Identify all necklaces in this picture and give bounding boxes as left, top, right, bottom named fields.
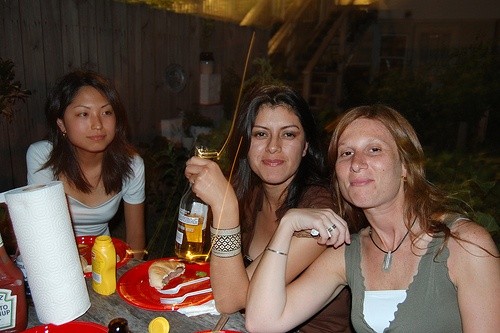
left=369, top=214, right=417, bottom=271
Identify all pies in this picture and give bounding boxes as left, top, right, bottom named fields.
left=148, top=261, right=185, bottom=289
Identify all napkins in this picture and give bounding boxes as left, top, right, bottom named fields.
left=177, top=299, right=221, bottom=317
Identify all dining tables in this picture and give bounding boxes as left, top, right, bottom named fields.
left=25, top=259, right=248, bottom=333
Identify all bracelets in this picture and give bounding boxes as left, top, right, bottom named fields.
left=210, top=225, right=242, bottom=258
left=266, top=247, right=288, bottom=256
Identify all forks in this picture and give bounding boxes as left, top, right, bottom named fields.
left=160, top=287, right=213, bottom=304
left=157, top=276, right=211, bottom=294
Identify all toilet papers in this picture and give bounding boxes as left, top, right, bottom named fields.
left=0, top=180, right=92, bottom=326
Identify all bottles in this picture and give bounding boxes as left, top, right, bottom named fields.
left=91, top=235, right=116, bottom=296
left=200, top=52, right=215, bottom=74
left=174, top=143, right=220, bottom=265
left=0, top=231, right=28, bottom=333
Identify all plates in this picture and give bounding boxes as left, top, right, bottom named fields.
left=21, top=321, right=109, bottom=333
left=116, top=257, right=213, bottom=311
left=75, top=235, right=132, bottom=278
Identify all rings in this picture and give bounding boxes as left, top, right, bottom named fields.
left=328, top=225, right=336, bottom=231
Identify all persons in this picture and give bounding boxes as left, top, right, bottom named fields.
left=246, top=102, right=500, bottom=333
left=184, top=84, right=337, bottom=313
left=26, top=70, right=147, bottom=258
